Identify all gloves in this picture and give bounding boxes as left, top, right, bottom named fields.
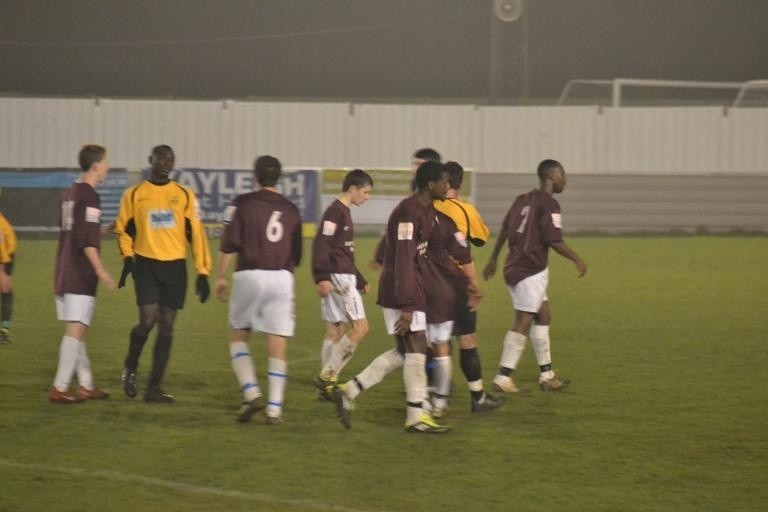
left=194, top=276, right=211, bottom=303
left=117, top=256, right=138, bottom=289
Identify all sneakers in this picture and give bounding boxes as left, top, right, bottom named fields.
left=471, top=392, right=507, bottom=416
left=491, top=375, right=530, bottom=395
left=48, top=385, right=86, bottom=406
left=333, top=383, right=359, bottom=430
left=429, top=391, right=449, bottom=418
left=121, top=366, right=139, bottom=398
left=404, top=414, right=453, bottom=434
left=312, top=373, right=340, bottom=401
left=76, top=385, right=109, bottom=401
left=2, top=336, right=15, bottom=344
left=143, top=388, right=175, bottom=404
left=539, top=376, right=571, bottom=392
left=263, top=411, right=287, bottom=427
left=234, top=393, right=266, bottom=425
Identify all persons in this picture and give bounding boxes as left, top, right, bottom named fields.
left=214, top=155, right=302, bottom=425
left=0, top=212, right=17, bottom=345
left=113, top=144, right=213, bottom=404
left=46, top=143, right=120, bottom=404
left=484, top=159, right=588, bottom=395
